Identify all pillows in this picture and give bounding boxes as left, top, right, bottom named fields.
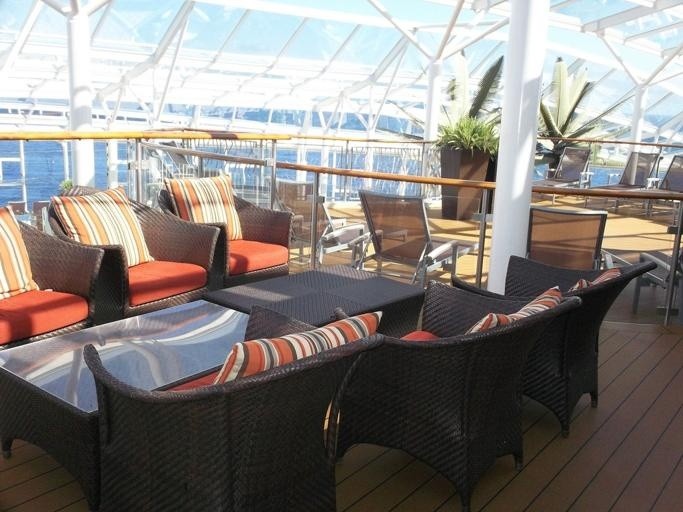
left=51, top=185, right=153, bottom=270
left=163, top=176, right=243, bottom=241
left=0, top=203, right=32, bottom=298
left=212, top=308, right=384, bottom=386
left=464, top=284, right=560, bottom=336
left=570, top=266, right=622, bottom=292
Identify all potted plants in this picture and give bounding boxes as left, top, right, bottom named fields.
left=434, top=111, right=500, bottom=220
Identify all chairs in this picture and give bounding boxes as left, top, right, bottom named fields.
left=532, top=146, right=594, bottom=202
left=0, top=221, right=105, bottom=350
left=644, top=155, right=683, bottom=220
left=584, top=152, right=662, bottom=213
left=48, top=191, right=222, bottom=323
left=273, top=184, right=363, bottom=268
left=359, top=188, right=478, bottom=284
left=453, top=256, right=658, bottom=424
left=159, top=183, right=293, bottom=287
left=340, top=278, right=579, bottom=512
left=631, top=200, right=683, bottom=324
left=85, top=301, right=381, bottom=512
left=528, top=207, right=613, bottom=273
